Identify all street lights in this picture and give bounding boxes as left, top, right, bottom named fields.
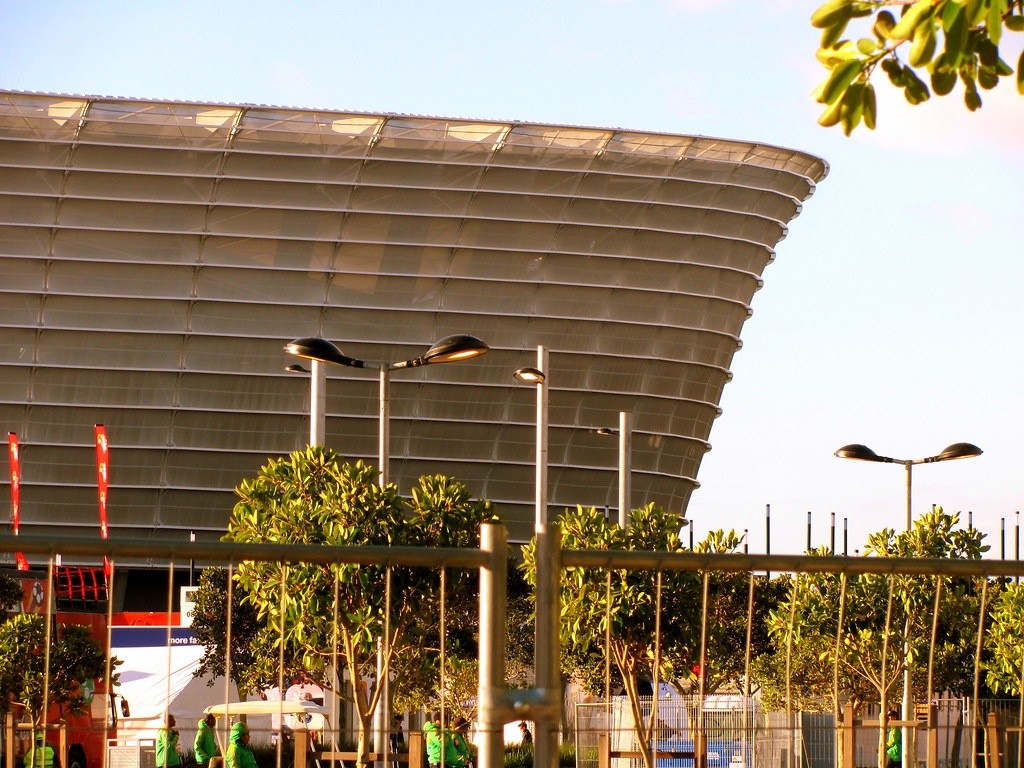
left=595, top=409, right=632, bottom=534
left=510, top=343, right=549, bottom=534
left=281, top=332, right=493, bottom=492
left=834, top=442, right=984, bottom=533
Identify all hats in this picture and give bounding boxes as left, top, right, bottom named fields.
left=887, top=711, right=897, bottom=716
left=36, top=734, right=43, bottom=740
left=451, top=717, right=467, bottom=731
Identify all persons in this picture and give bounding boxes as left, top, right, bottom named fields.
left=886, top=711, right=902, bottom=768
left=156, top=714, right=182, bottom=768
left=423, top=712, right=475, bottom=768
left=226, top=722, right=257, bottom=768
left=390, top=715, right=407, bottom=753
left=517, top=722, right=532, bottom=746
left=24, top=734, right=54, bottom=768
left=194, top=714, right=216, bottom=768
